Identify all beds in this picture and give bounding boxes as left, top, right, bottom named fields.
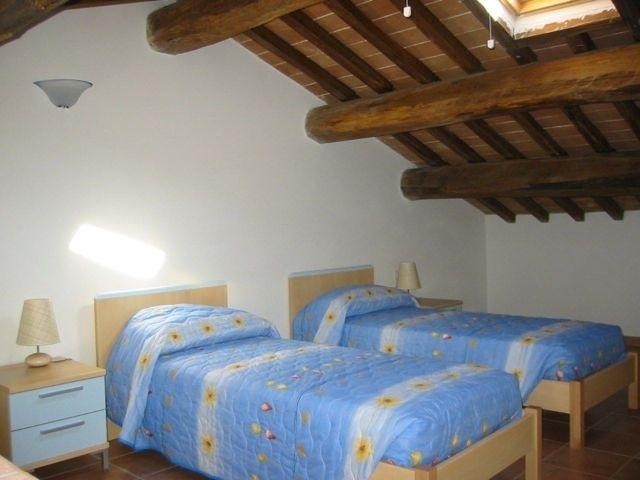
left=94, top=280, right=543, bottom=480
left=289, top=265, right=639, bottom=450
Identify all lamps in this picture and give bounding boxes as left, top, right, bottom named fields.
left=16, top=298, right=60, bottom=367
left=33, top=79, right=93, bottom=109
left=396, top=262, right=421, bottom=293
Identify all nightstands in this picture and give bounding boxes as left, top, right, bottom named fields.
left=416, top=298, right=463, bottom=311
left=0, top=357, right=110, bottom=472
